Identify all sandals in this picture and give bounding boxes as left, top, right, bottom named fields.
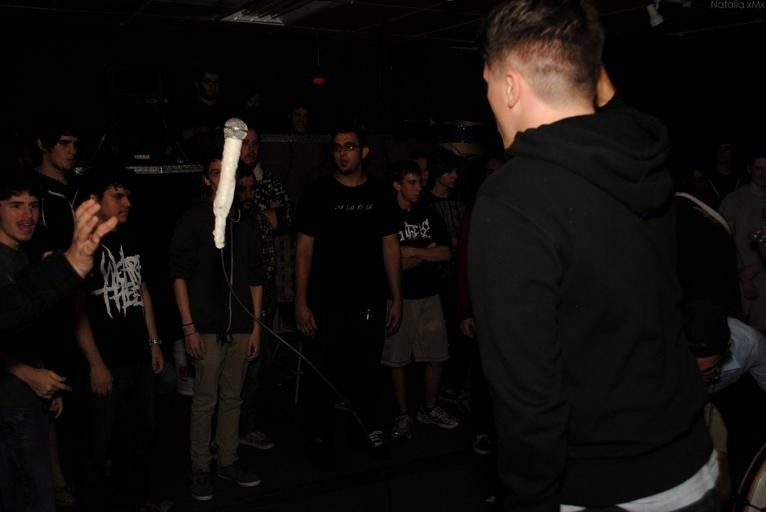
left=51, top=482, right=75, bottom=507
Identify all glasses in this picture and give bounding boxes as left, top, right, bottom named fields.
left=331, top=141, right=363, bottom=153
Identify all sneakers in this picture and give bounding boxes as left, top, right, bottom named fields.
left=439, top=388, right=459, bottom=404
left=209, top=441, right=239, bottom=464
left=190, top=468, right=214, bottom=501
left=416, top=405, right=460, bottom=429
left=239, top=432, right=274, bottom=450
left=390, top=413, right=413, bottom=440
left=215, top=460, right=262, bottom=487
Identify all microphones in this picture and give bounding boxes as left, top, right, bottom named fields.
left=213, top=118, right=248, bottom=250
